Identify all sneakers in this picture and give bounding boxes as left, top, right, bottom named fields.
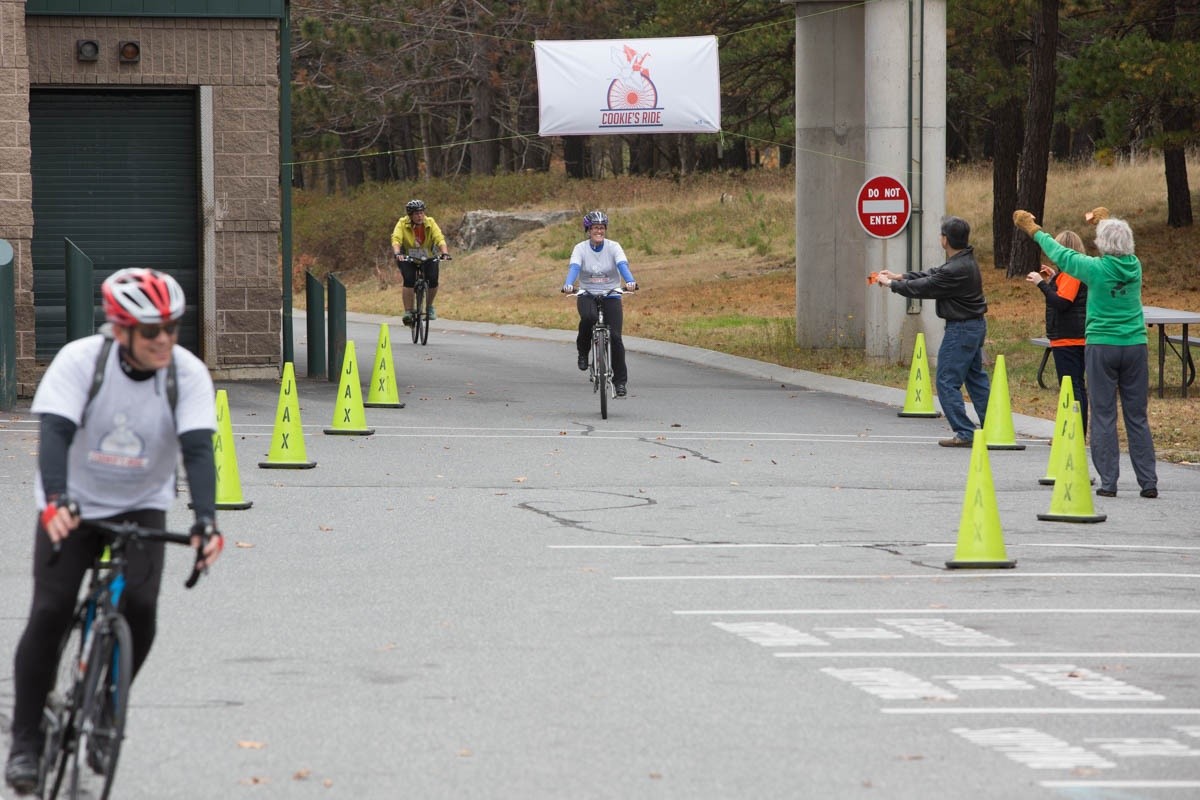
left=86, top=726, right=117, bottom=776
left=6, top=735, right=40, bottom=786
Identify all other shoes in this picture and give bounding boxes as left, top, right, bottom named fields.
left=616, top=383, right=627, bottom=399
left=426, top=306, right=435, bottom=319
left=578, top=351, right=588, bottom=371
left=1095, top=489, right=1115, bottom=497
left=401, top=313, right=413, bottom=326
left=939, top=437, right=973, bottom=448
left=1141, top=487, right=1158, bottom=498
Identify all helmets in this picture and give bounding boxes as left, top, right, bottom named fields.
left=583, top=211, right=608, bottom=226
left=101, top=267, right=186, bottom=325
left=405, top=200, right=426, bottom=211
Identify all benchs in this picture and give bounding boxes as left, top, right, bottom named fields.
left=1165, top=335, right=1200, bottom=387
left=1031, top=338, right=1051, bottom=389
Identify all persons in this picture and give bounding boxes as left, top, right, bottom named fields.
left=1025, top=230, right=1088, bottom=446
left=876, top=216, right=990, bottom=447
left=4, top=266, right=225, bottom=792
left=390, top=200, right=450, bottom=326
left=1012, top=207, right=1159, bottom=498
left=562, top=211, right=636, bottom=396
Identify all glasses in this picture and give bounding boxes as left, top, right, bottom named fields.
left=116, top=323, right=182, bottom=339
left=589, top=226, right=606, bottom=232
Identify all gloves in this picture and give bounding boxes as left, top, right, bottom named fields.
left=1014, top=210, right=1041, bottom=237
left=1089, top=207, right=1111, bottom=224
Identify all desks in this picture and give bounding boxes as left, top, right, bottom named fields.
left=1141, top=305, right=1200, bottom=399
